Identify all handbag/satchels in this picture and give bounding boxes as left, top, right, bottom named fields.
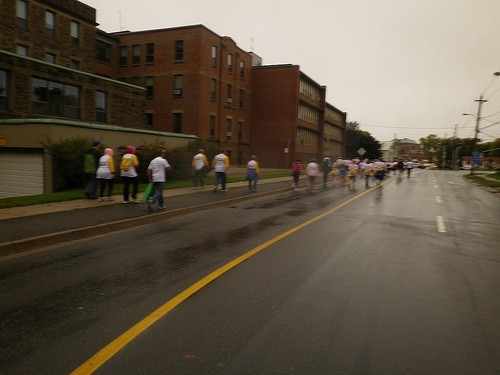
left=293, top=171, right=299, bottom=178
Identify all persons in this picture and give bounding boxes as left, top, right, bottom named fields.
left=147, top=150, right=171, bottom=210
left=246, top=155, right=259, bottom=192
left=83, top=139, right=103, bottom=200
left=212, top=148, right=230, bottom=192
left=192, top=148, right=209, bottom=190
left=322, top=156, right=413, bottom=191
left=119, top=146, right=140, bottom=204
left=291, top=159, right=302, bottom=187
left=96, top=147, right=116, bottom=202
left=305, top=159, right=319, bottom=190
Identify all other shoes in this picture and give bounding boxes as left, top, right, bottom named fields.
left=98, top=199, right=103, bottom=202
left=221, top=189, right=229, bottom=192
left=249, top=190, right=251, bottom=192
left=85, top=192, right=91, bottom=199
left=108, top=197, right=113, bottom=200
left=131, top=197, right=136, bottom=202
left=123, top=201, right=129, bottom=203
left=157, top=206, right=166, bottom=209
left=148, top=200, right=154, bottom=210
left=252, top=189, right=256, bottom=191
left=92, top=196, right=98, bottom=199
left=201, top=186, right=206, bottom=188
left=193, top=186, right=199, bottom=190
left=213, top=186, right=218, bottom=192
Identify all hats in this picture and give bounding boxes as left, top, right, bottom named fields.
left=93, top=140, right=100, bottom=146
left=127, top=146, right=137, bottom=154
left=104, top=148, right=112, bottom=155
